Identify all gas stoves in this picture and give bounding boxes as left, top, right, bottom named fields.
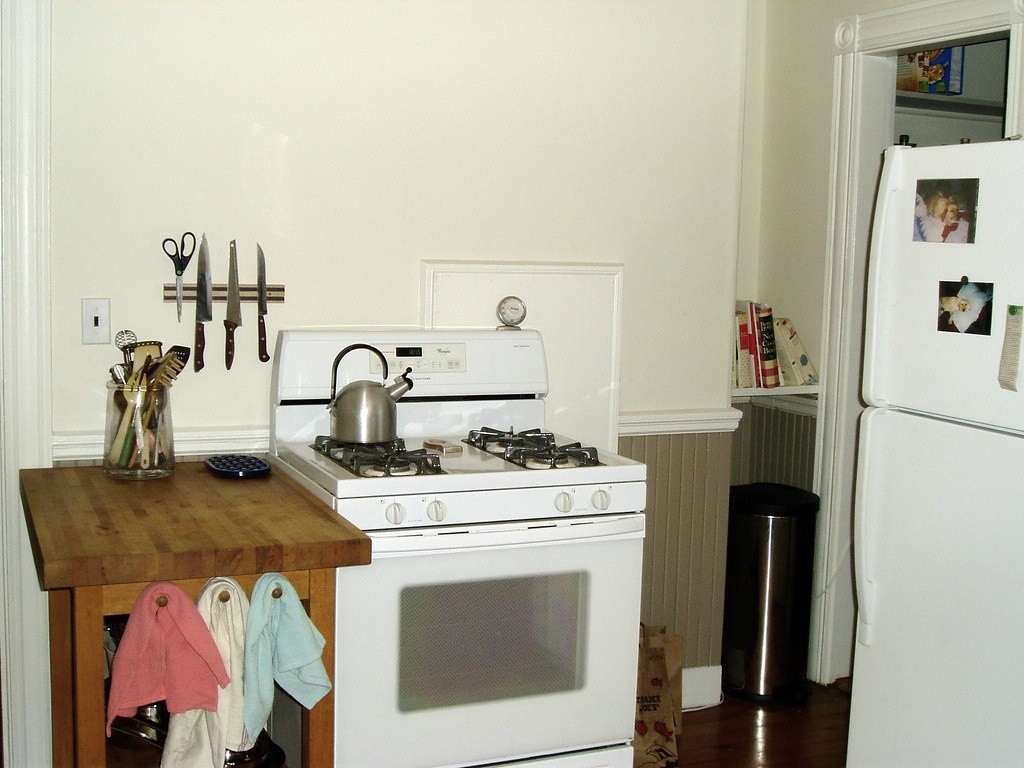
left=269, top=329, right=647, bottom=530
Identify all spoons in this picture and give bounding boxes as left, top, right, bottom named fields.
left=153, top=389, right=167, bottom=467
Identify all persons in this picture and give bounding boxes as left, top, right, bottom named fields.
left=915, top=190, right=968, bottom=243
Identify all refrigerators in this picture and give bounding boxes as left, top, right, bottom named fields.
left=846, top=139, right=1024, bottom=768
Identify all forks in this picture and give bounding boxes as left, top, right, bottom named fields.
left=108, top=371, right=147, bottom=465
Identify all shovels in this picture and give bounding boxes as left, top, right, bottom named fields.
left=117, top=340, right=190, bottom=466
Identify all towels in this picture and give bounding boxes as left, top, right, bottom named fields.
left=106, top=573, right=331, bottom=768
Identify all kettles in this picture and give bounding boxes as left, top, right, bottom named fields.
left=325, top=344, right=413, bottom=445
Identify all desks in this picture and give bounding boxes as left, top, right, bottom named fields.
left=19, top=461, right=372, bottom=768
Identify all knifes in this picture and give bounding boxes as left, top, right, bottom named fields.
left=257, top=243, right=270, bottom=363
left=194, top=232, right=213, bottom=373
left=223, top=239, right=242, bottom=370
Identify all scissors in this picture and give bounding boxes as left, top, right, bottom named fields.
left=162, top=232, right=197, bottom=324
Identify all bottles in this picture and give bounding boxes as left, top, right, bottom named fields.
left=102, top=380, right=175, bottom=483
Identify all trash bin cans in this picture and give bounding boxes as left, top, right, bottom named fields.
left=724, top=482, right=820, bottom=705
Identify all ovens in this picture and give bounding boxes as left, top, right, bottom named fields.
left=336, top=513, right=645, bottom=768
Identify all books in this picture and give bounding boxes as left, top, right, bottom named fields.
left=896, top=46, right=965, bottom=95
left=732, top=302, right=818, bottom=388
